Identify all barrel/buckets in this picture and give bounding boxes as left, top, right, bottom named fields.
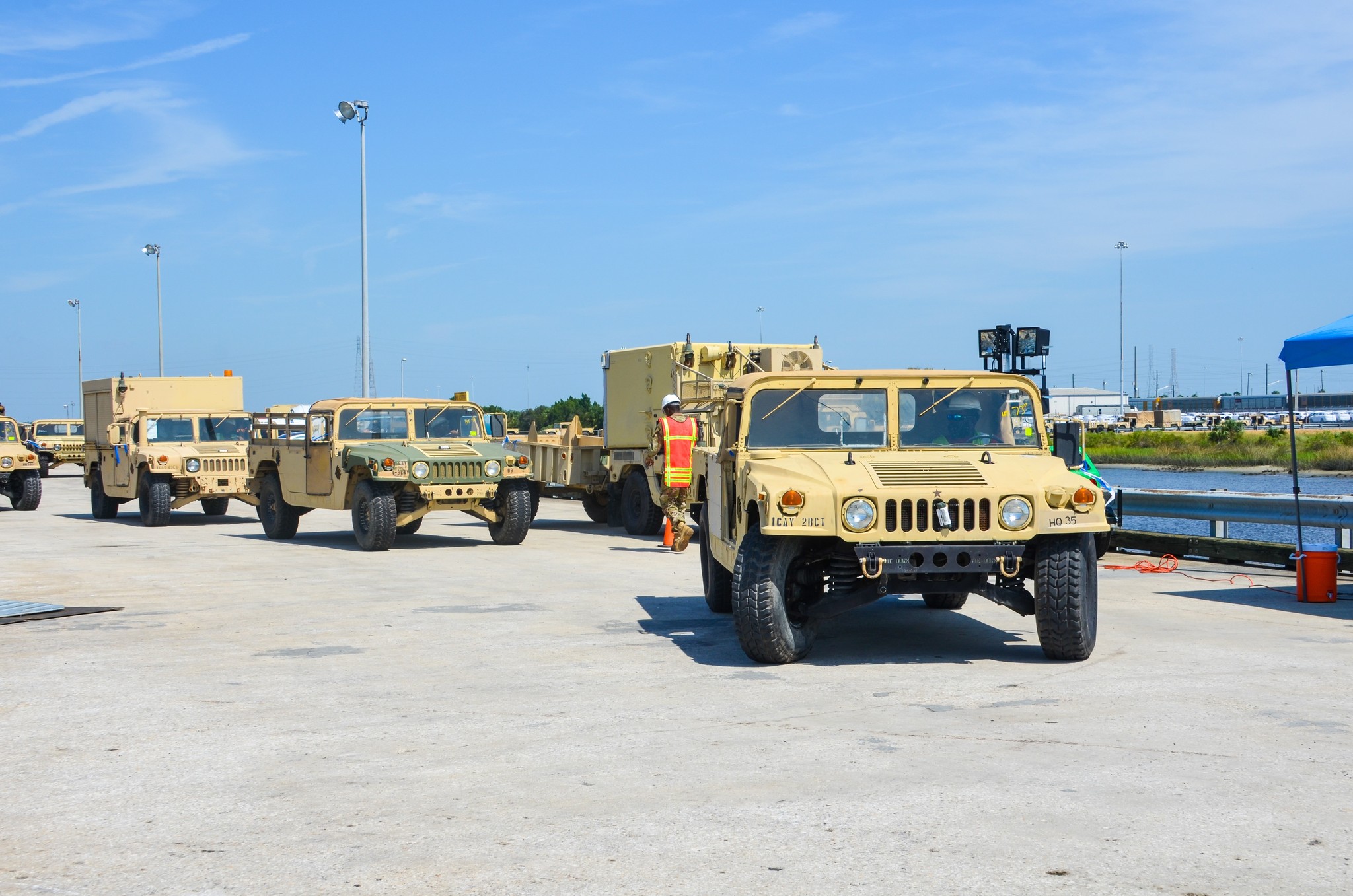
left=1289, top=543, right=1341, bottom=602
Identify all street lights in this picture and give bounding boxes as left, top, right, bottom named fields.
left=334, top=99, right=370, bottom=403
left=755, top=307, right=764, bottom=344
left=425, top=389, right=429, bottom=398
left=1053, top=385, right=1058, bottom=389
left=63, top=405, right=69, bottom=419
left=1247, top=371, right=1254, bottom=395
left=1104, top=379, right=1107, bottom=391
left=1114, top=240, right=1129, bottom=413
left=1320, top=369, right=1326, bottom=391
left=67, top=299, right=82, bottom=415
left=399, top=358, right=407, bottom=399
left=436, top=386, right=440, bottom=399
left=470, top=377, right=475, bottom=401
left=1237, top=336, right=1245, bottom=395
left=141, top=243, right=165, bottom=378
left=1204, top=366, right=1209, bottom=400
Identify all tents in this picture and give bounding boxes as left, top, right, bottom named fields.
left=1277, top=315, right=1353, bottom=602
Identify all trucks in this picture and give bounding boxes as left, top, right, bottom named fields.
left=0, top=402, right=42, bottom=512
left=25, top=418, right=85, bottom=478
left=689, top=366, right=1112, bottom=665
left=491, top=333, right=824, bottom=538
left=80, top=370, right=272, bottom=529
left=242, top=398, right=538, bottom=553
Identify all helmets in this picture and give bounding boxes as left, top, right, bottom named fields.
left=224, top=418, right=236, bottom=426
left=944, top=392, right=982, bottom=411
left=431, top=416, right=449, bottom=429
left=661, top=394, right=681, bottom=412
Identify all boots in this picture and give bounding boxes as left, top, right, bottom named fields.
left=670, top=522, right=694, bottom=552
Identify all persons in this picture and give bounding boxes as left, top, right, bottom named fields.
left=430, top=415, right=460, bottom=438
left=644, top=394, right=698, bottom=552
left=363, top=421, right=390, bottom=433
left=212, top=417, right=244, bottom=440
left=930, top=392, right=1002, bottom=446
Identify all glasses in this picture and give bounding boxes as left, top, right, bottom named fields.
left=662, top=411, right=666, bottom=415
left=947, top=414, right=976, bottom=421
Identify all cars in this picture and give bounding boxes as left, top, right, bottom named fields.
left=1012, top=410, right=1353, bottom=429
